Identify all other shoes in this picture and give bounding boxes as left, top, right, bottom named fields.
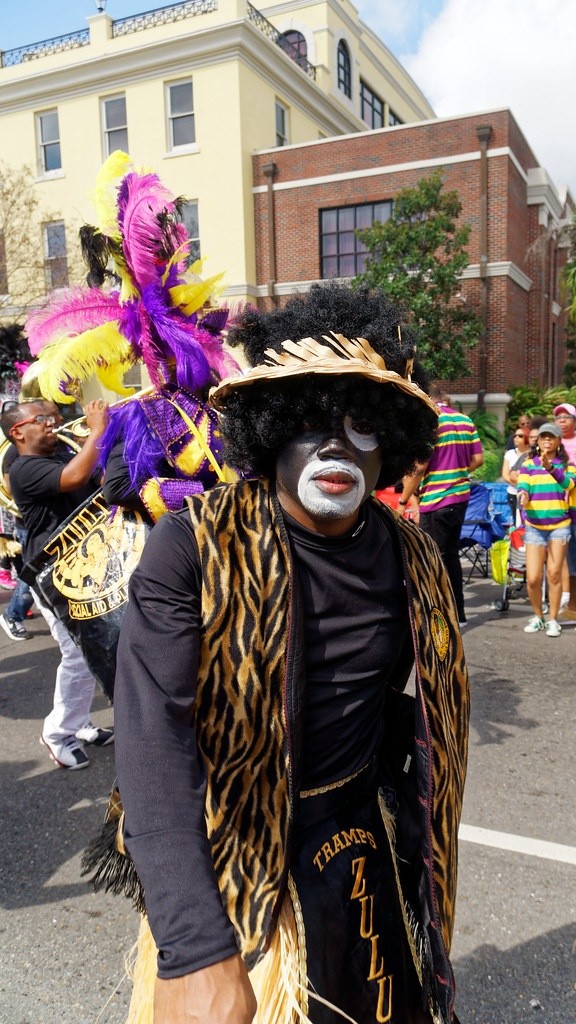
left=557, top=606, right=576, bottom=624
left=459, top=616, right=469, bottom=628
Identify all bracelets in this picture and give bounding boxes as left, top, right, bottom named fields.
left=398, top=497, right=408, bottom=505
left=546, top=464, right=554, bottom=472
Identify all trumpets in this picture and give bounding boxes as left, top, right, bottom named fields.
left=49, top=368, right=167, bottom=439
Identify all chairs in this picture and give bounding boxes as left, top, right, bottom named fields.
left=459, top=482, right=490, bottom=584
left=487, top=483, right=515, bottom=529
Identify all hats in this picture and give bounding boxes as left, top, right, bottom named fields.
left=206, top=292, right=440, bottom=489
left=537, top=423, right=562, bottom=442
left=552, top=402, right=576, bottom=419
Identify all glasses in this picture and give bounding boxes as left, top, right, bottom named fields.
left=552, top=415, right=575, bottom=420
left=514, top=433, right=525, bottom=438
left=7, top=414, right=54, bottom=435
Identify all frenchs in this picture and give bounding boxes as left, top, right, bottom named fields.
left=0, top=332, right=82, bottom=519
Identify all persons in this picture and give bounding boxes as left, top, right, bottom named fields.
left=502, top=403, right=576, bottom=636
left=398, top=383, right=483, bottom=626
left=0, top=398, right=115, bottom=770
left=81, top=284, right=471, bottom=1024
left=0, top=400, right=34, bottom=641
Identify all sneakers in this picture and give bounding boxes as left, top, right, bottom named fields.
left=38, top=732, right=89, bottom=770
left=524, top=617, right=543, bottom=633
left=75, top=719, right=115, bottom=745
left=0, top=611, right=30, bottom=640
left=545, top=619, right=560, bottom=637
left=0, top=569, right=17, bottom=590
